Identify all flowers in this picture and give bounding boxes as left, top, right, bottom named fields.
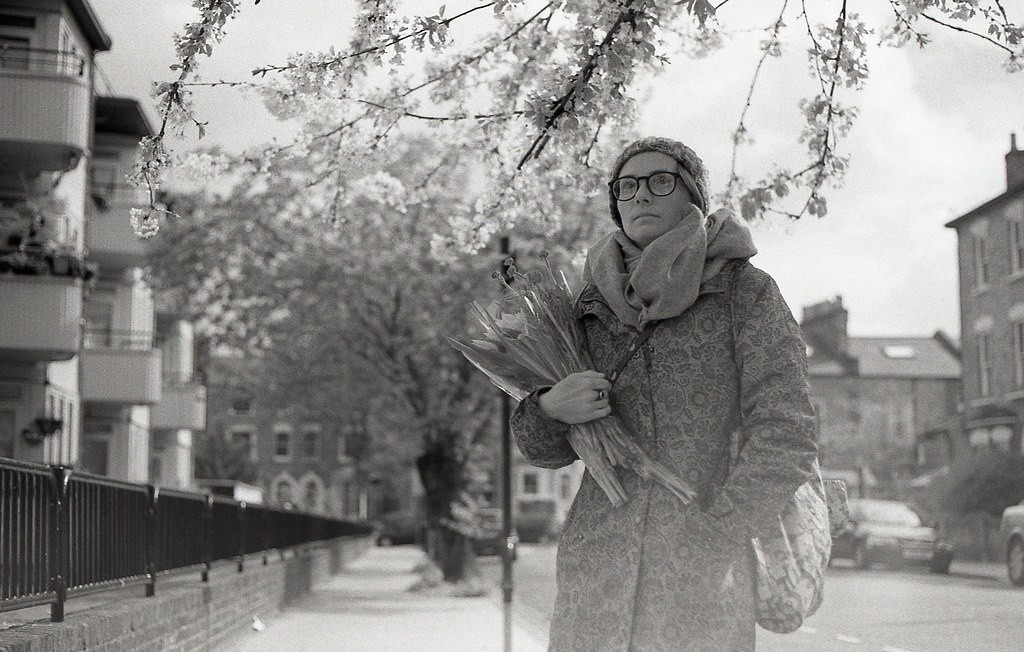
left=443, top=250, right=698, bottom=505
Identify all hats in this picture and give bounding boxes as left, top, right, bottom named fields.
left=608, top=136, right=710, bottom=229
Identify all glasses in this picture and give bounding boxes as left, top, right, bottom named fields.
left=612, top=171, right=680, bottom=201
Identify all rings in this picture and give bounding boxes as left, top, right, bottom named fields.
left=595, top=389, right=605, bottom=401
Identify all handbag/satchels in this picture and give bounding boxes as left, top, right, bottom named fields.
left=730, top=424, right=832, bottom=635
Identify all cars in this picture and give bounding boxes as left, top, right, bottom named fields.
left=377, top=513, right=430, bottom=548
left=999, top=500, right=1024, bottom=586
left=473, top=509, right=517, bottom=562
left=829, top=499, right=953, bottom=573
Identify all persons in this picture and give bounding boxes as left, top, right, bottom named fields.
left=510, top=136, right=818, bottom=650
